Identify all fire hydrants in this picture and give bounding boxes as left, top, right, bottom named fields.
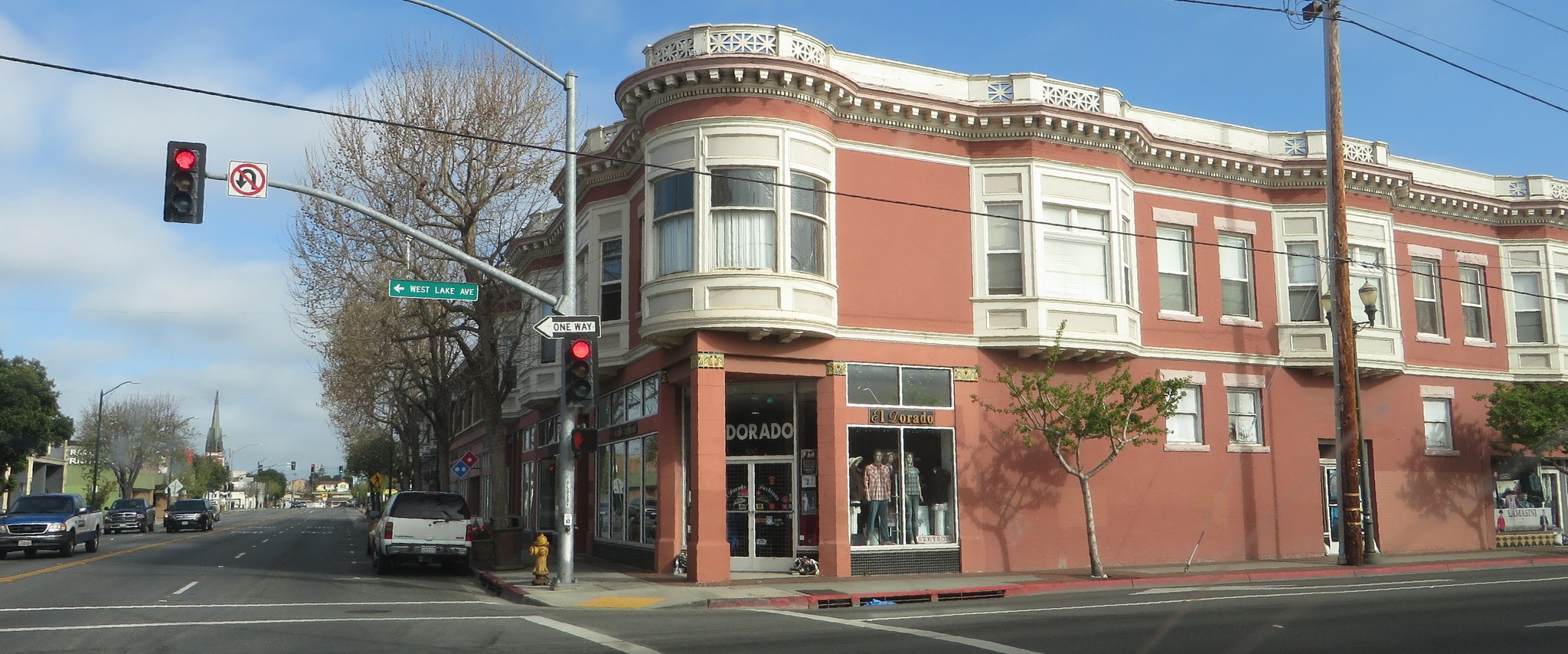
left=528, top=532, right=550, bottom=586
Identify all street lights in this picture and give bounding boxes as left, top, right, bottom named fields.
left=207, top=435, right=230, bottom=501
left=90, top=380, right=142, bottom=512
left=1317, top=277, right=1382, bottom=566
left=166, top=416, right=202, bottom=508
left=228, top=443, right=263, bottom=511
left=255, top=457, right=270, bottom=510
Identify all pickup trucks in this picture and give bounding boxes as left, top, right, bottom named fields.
left=0, top=492, right=105, bottom=562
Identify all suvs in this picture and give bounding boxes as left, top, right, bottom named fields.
left=103, top=496, right=156, bottom=535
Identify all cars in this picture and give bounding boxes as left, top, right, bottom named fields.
left=366, top=489, right=473, bottom=576
left=289, top=500, right=307, bottom=509
left=163, top=497, right=221, bottom=533
left=344, top=500, right=358, bottom=507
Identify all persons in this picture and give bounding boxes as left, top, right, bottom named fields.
left=1497, top=509, right=1505, bottom=532
left=1540, top=509, right=1549, bottom=529
left=1518, top=493, right=1533, bottom=507
left=849, top=448, right=923, bottom=545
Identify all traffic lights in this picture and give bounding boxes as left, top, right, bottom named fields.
left=571, top=428, right=598, bottom=452
left=311, top=464, right=315, bottom=472
left=570, top=339, right=594, bottom=400
left=163, top=141, right=207, bottom=225
left=339, top=465, right=343, bottom=473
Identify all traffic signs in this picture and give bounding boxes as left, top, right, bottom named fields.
left=532, top=314, right=602, bottom=340
